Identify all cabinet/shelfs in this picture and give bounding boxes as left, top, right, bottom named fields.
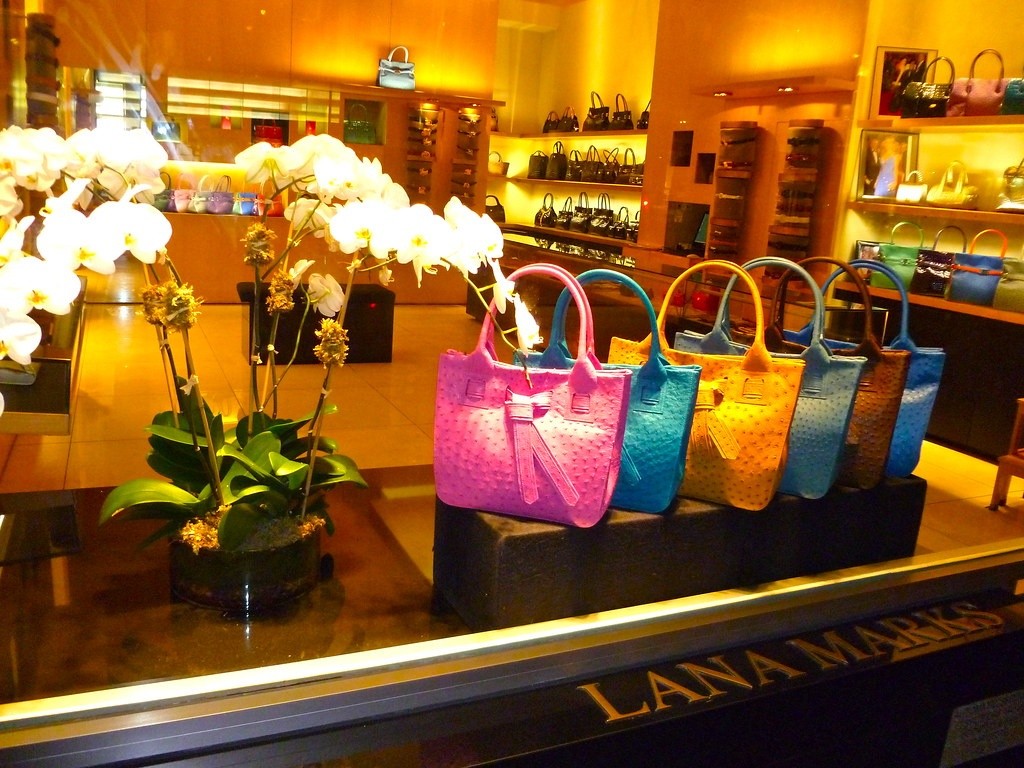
left=832, top=114, right=1024, bottom=465
left=497, top=129, right=649, bottom=250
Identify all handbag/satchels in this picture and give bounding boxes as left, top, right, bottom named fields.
left=155, top=171, right=285, bottom=217
left=997, top=158, right=1024, bottom=210
left=528, top=91, right=651, bottom=243
left=925, top=160, right=979, bottom=210
left=253, top=119, right=283, bottom=148
left=764, top=256, right=910, bottom=489
left=675, top=257, right=867, bottom=499
left=380, top=45, right=416, bottom=91
left=900, top=56, right=956, bottom=117
left=784, top=259, right=945, bottom=480
left=485, top=195, right=505, bottom=222
left=517, top=269, right=702, bottom=513
left=947, top=49, right=1012, bottom=116
left=434, top=263, right=633, bottom=527
left=344, top=103, right=375, bottom=144
left=608, top=260, right=806, bottom=511
left=895, top=171, right=928, bottom=205
left=1001, top=66, right=1024, bottom=114
left=871, top=220, right=1023, bottom=307
left=488, top=151, right=509, bottom=175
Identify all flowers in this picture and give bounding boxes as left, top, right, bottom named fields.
left=0, top=117, right=544, bottom=565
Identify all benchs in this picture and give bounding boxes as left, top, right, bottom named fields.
left=236, top=282, right=395, bottom=367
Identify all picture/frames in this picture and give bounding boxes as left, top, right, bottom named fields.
left=855, top=129, right=920, bottom=204
left=867, top=46, right=938, bottom=119
left=855, top=239, right=895, bottom=284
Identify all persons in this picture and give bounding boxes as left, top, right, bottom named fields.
left=863, top=134, right=907, bottom=197
left=882, top=54, right=926, bottom=112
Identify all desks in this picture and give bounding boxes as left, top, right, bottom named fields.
left=430, top=473, right=927, bottom=629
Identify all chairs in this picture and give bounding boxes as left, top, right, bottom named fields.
left=988, top=397, right=1024, bottom=511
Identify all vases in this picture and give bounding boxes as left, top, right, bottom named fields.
left=167, top=509, right=324, bottom=613
